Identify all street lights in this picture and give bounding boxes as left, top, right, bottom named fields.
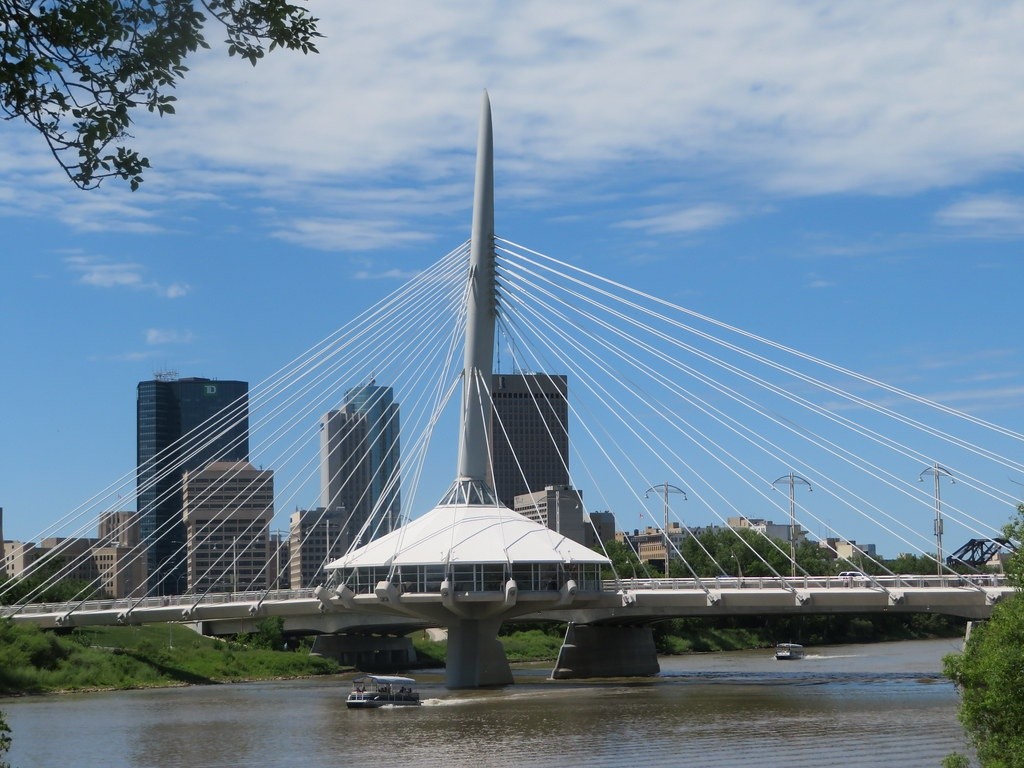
left=644, top=482, right=687, bottom=579
left=918, top=463, right=956, bottom=575
left=771, top=472, right=813, bottom=581
left=212, top=535, right=255, bottom=602
left=536, top=490, right=580, bottom=590
left=255, top=528, right=301, bottom=590
left=305, top=518, right=351, bottom=583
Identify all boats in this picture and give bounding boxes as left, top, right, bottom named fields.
left=774, top=643, right=804, bottom=659
left=344, top=675, right=422, bottom=708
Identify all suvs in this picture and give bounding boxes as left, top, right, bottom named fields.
left=838, top=572, right=873, bottom=587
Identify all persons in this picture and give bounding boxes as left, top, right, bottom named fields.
left=355, top=687, right=362, bottom=692
left=361, top=686, right=367, bottom=693
left=386, top=684, right=412, bottom=694
